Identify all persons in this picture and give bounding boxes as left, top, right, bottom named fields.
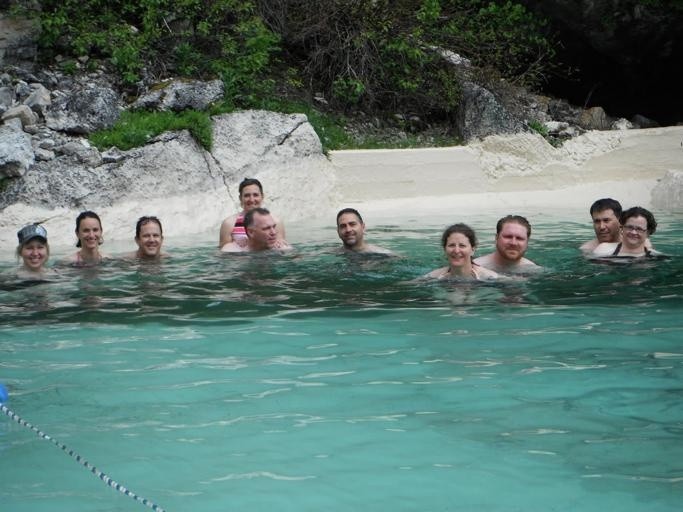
left=3, top=223, right=58, bottom=277
left=220, top=177, right=285, bottom=243
left=126, top=214, right=170, bottom=261
left=318, top=209, right=391, bottom=251
left=579, top=197, right=623, bottom=251
left=473, top=214, right=536, bottom=270
left=222, top=207, right=290, bottom=251
left=597, top=206, right=662, bottom=256
left=413, top=224, right=500, bottom=280
left=56, top=210, right=112, bottom=265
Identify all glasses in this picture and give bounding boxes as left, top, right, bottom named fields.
left=139, top=216, right=159, bottom=225
left=625, top=224, right=648, bottom=233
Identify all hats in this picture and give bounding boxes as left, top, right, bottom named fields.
left=17, top=225, right=48, bottom=244
